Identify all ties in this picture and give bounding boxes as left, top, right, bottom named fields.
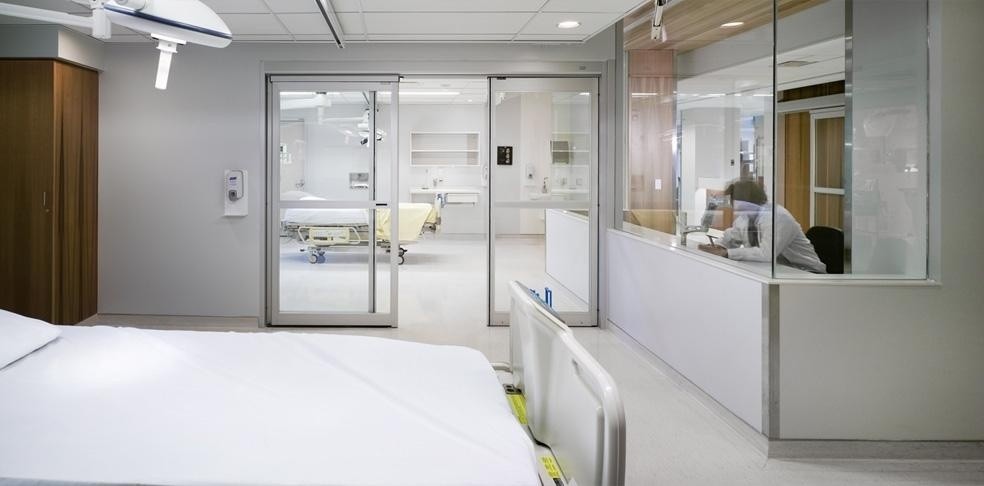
left=748, top=219, right=760, bottom=248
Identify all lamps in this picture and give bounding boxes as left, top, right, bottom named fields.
left=77, top=0, right=237, bottom=92
left=353, top=119, right=383, bottom=149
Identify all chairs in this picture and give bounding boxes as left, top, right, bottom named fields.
left=784, top=223, right=845, bottom=275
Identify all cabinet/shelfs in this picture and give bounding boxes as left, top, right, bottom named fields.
left=0, top=58, right=100, bottom=325
left=406, top=129, right=484, bottom=172
left=550, top=130, right=590, bottom=167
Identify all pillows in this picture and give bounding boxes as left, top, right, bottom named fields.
left=0, top=308, right=63, bottom=372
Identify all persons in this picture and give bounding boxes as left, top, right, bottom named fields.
left=698, top=182, right=828, bottom=274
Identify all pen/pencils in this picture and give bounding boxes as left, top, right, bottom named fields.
left=709, top=235, right=715, bottom=246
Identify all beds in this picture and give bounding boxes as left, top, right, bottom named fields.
left=1, top=278, right=631, bottom=486
left=278, top=187, right=439, bottom=264
